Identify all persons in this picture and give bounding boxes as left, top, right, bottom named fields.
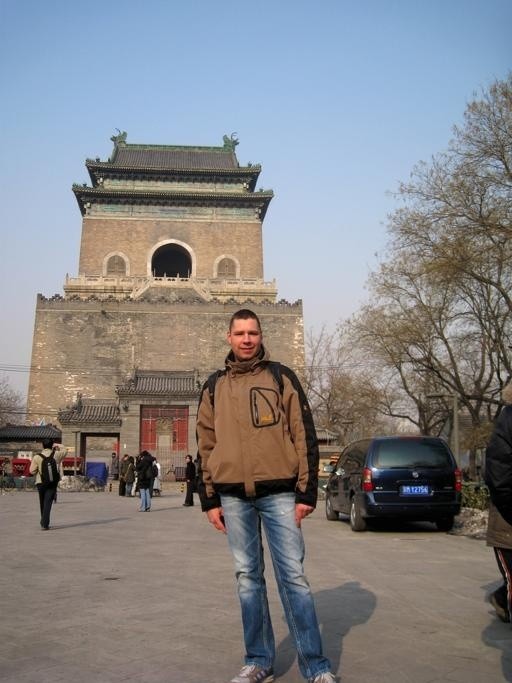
left=29, top=437, right=67, bottom=530
left=111, top=452, right=120, bottom=479
left=197, top=309, right=339, bottom=683
left=183, top=454, right=195, bottom=506
left=486, top=378, right=512, bottom=622
left=118, top=449, right=162, bottom=512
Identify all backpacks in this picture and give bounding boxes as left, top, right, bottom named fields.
left=36, top=448, right=59, bottom=487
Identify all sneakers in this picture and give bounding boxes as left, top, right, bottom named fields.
left=39, top=524, right=50, bottom=530
left=488, top=590, right=510, bottom=622
left=308, top=672, right=337, bottom=683
left=118, top=493, right=195, bottom=513
left=230, top=663, right=274, bottom=683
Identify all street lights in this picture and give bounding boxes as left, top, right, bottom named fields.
left=427, top=393, right=460, bottom=468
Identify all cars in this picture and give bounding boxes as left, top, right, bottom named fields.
left=326, top=435, right=463, bottom=532
